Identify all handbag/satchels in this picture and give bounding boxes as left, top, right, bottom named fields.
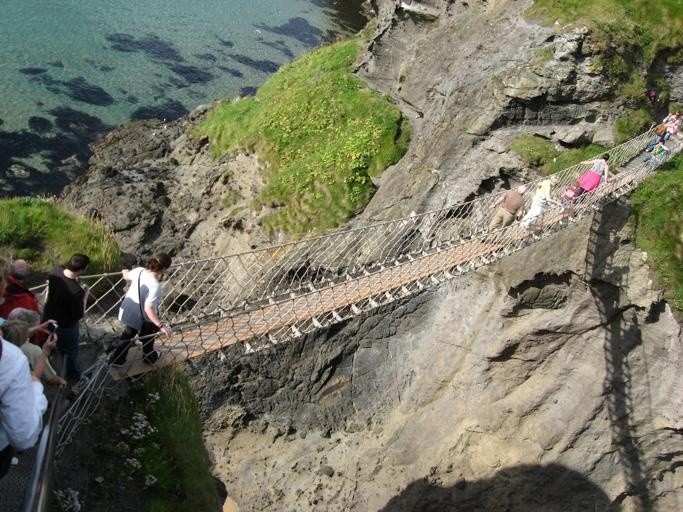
left=654, top=123, right=667, bottom=136
left=139, top=321, right=158, bottom=344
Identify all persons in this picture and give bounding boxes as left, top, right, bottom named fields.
left=520, top=176, right=563, bottom=229
left=480, top=184, right=527, bottom=244
left=0, top=259, right=68, bottom=479
left=557, top=183, right=579, bottom=214
left=572, top=153, right=610, bottom=204
left=643, top=110, right=683, bottom=171
left=110, top=253, right=173, bottom=366
left=40, top=254, right=91, bottom=379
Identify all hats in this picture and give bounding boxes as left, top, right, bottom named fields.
left=518, top=185, right=527, bottom=193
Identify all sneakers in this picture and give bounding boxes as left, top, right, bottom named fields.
left=156, top=350, right=162, bottom=360
left=76, top=376, right=89, bottom=387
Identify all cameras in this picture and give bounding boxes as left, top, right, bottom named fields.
left=48, top=322, right=58, bottom=333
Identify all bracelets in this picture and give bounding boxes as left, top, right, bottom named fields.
left=157, top=323, right=163, bottom=328
